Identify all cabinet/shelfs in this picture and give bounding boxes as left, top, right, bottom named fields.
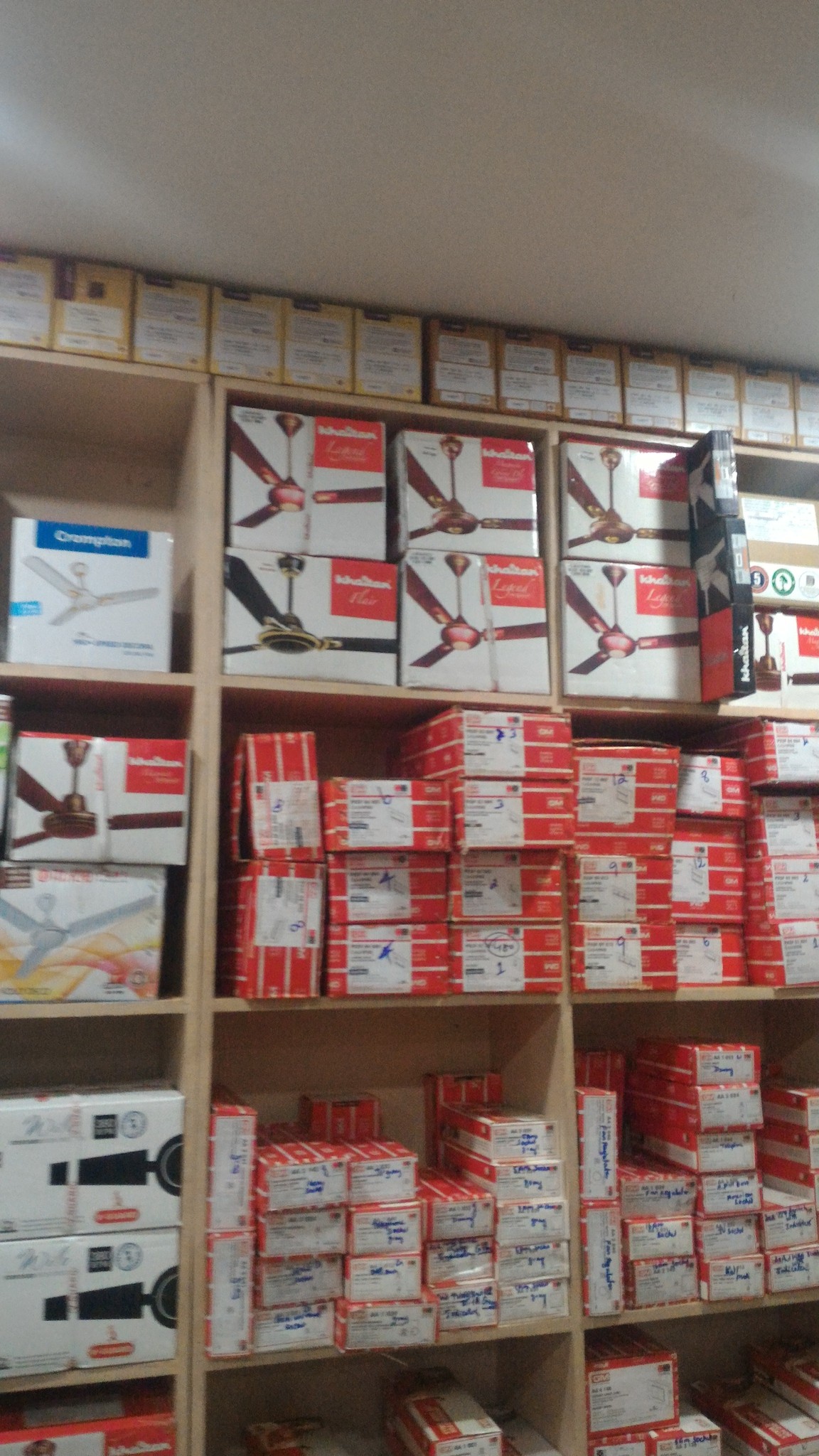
left=0, top=342, right=819, bottom=1456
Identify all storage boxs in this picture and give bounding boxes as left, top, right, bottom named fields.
left=0, top=245, right=819, bottom=1456
